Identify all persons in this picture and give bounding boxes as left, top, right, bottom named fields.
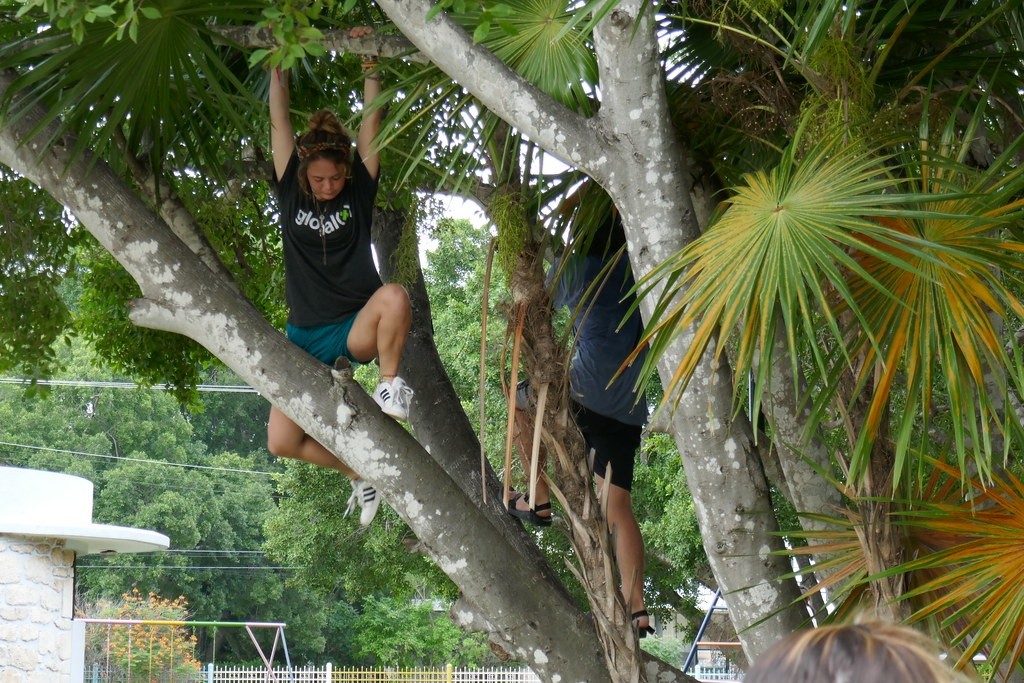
left=265, top=20, right=414, bottom=530
left=741, top=623, right=980, bottom=683
left=498, top=177, right=655, bottom=639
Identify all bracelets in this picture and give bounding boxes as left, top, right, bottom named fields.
left=360, top=53, right=380, bottom=67
left=364, top=75, right=382, bottom=81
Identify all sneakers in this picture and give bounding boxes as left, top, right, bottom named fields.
left=350, top=474, right=383, bottom=528
left=371, top=377, right=414, bottom=421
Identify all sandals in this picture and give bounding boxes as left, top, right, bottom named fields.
left=495, top=484, right=554, bottom=525
left=628, top=608, right=656, bottom=638
